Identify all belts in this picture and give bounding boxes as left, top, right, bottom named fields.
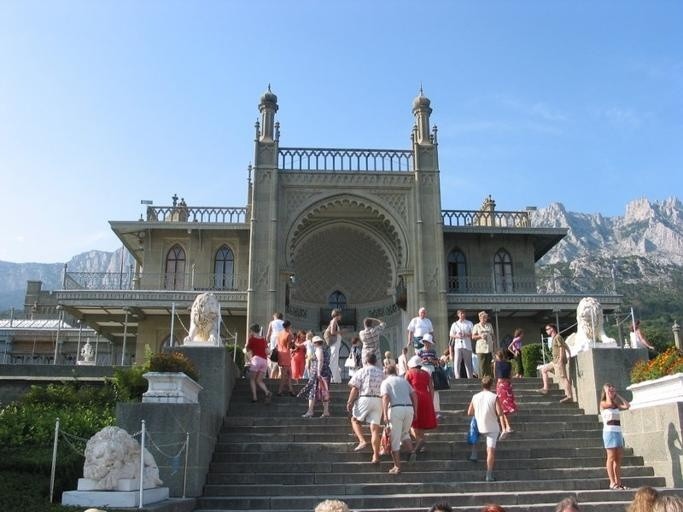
left=360, top=395, right=382, bottom=399
left=389, top=404, right=413, bottom=408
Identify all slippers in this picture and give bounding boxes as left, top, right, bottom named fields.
left=620, top=483, right=629, bottom=490
left=610, top=484, right=622, bottom=490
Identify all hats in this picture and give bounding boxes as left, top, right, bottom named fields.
left=407, top=355, right=423, bottom=368
left=312, top=335, right=323, bottom=343
left=419, top=333, right=433, bottom=344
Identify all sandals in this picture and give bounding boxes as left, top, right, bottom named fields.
left=252, top=388, right=329, bottom=418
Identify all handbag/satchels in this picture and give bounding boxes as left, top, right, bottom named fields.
left=270, top=347, right=278, bottom=362
left=344, top=357, right=356, bottom=368
left=468, top=416, right=480, bottom=445
left=431, top=367, right=450, bottom=390
left=324, top=320, right=337, bottom=346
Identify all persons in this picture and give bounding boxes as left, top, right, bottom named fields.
left=245, top=308, right=387, bottom=418
left=407, top=307, right=525, bottom=379
left=598, top=381, right=632, bottom=490
left=346, top=348, right=438, bottom=473
left=465, top=375, right=507, bottom=482
left=308, top=485, right=682, bottom=512
left=627, top=318, right=656, bottom=350
left=535, top=323, right=573, bottom=403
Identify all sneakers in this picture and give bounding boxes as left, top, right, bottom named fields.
left=354, top=437, right=425, bottom=475
left=469, top=430, right=513, bottom=481
